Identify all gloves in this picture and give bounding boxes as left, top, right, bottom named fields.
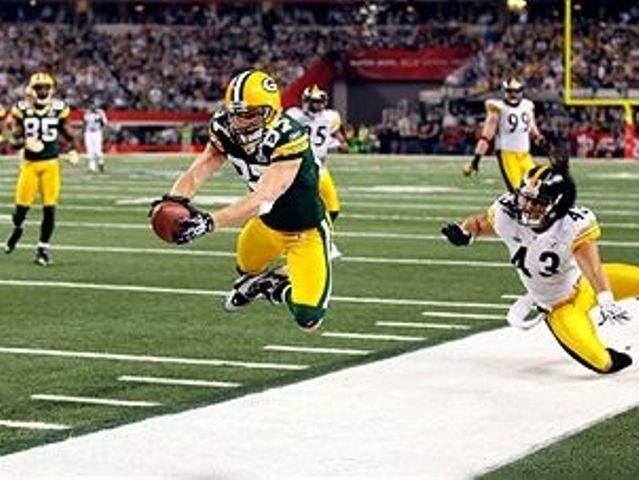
left=147, top=193, right=214, bottom=245
left=441, top=221, right=475, bottom=246
left=597, top=291, right=631, bottom=326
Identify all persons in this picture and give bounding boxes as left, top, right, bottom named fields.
left=280, top=85, right=354, bottom=262
left=462, top=75, right=567, bottom=193
left=148, top=68, right=333, bottom=332
left=438, top=161, right=638, bottom=373
left=77, top=98, right=109, bottom=178
left=2, top=72, right=82, bottom=267
left=1, top=0, right=638, bottom=160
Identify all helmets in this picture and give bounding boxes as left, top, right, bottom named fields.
left=301, top=84, right=329, bottom=120
left=225, top=69, right=281, bottom=155
left=513, top=165, right=576, bottom=229
left=25, top=73, right=58, bottom=106
left=502, top=74, right=526, bottom=105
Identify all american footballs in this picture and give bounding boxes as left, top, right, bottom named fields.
left=152, top=203, right=191, bottom=242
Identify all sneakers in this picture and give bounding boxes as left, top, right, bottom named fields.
left=5, top=226, right=23, bottom=253
left=33, top=247, right=51, bottom=266
left=224, top=265, right=271, bottom=311
